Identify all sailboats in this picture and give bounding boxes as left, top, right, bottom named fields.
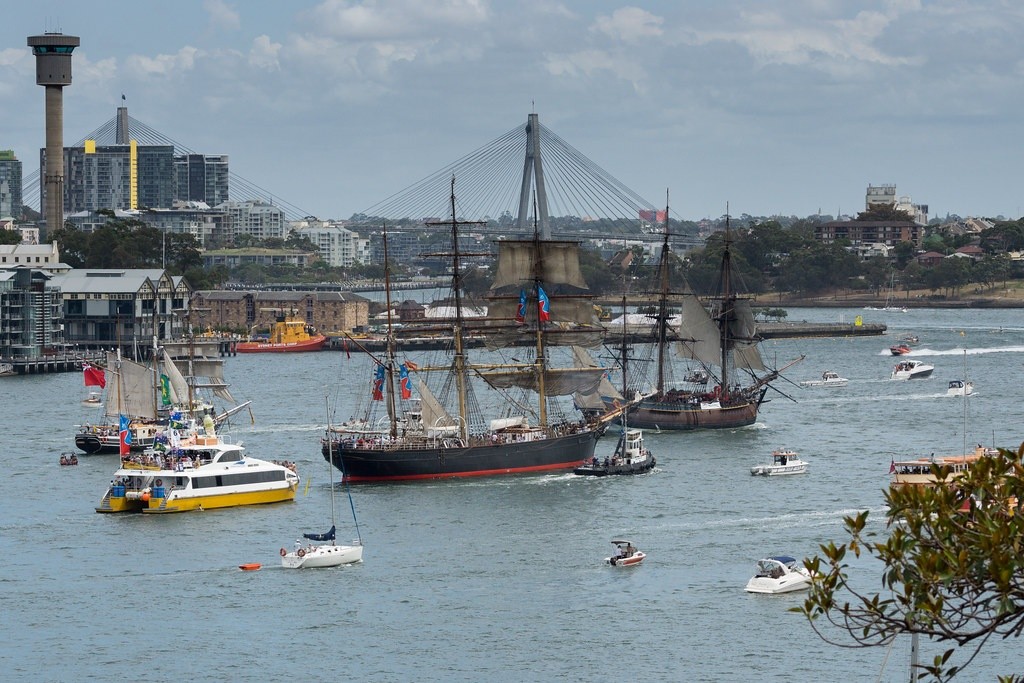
left=574, top=201, right=809, bottom=435
left=277, top=392, right=365, bottom=570
left=93, top=306, right=301, bottom=516
left=883, top=269, right=909, bottom=314
left=320, top=169, right=665, bottom=489
left=73, top=303, right=257, bottom=456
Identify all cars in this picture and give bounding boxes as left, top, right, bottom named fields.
left=379, top=325, right=388, bottom=334
left=368, top=325, right=377, bottom=334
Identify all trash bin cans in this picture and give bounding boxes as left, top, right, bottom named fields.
left=113, top=486, right=126, bottom=497
left=353, top=326, right=363, bottom=332
left=153, top=487, right=165, bottom=499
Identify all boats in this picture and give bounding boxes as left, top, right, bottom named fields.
left=890, top=358, right=936, bottom=381
left=889, top=341, right=914, bottom=357
left=800, top=369, right=851, bottom=388
left=230, top=303, right=328, bottom=354
left=603, top=539, right=648, bottom=568
left=944, top=379, right=974, bottom=397
left=743, top=556, right=821, bottom=595
left=748, top=445, right=811, bottom=478
left=895, top=332, right=921, bottom=344
left=888, top=443, right=1022, bottom=516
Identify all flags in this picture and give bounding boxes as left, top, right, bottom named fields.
left=889, top=458, right=895, bottom=474
left=81, top=362, right=106, bottom=389
left=153, top=411, right=182, bottom=452
left=405, top=360, right=418, bottom=370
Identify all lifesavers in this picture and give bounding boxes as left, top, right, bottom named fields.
left=280, top=548, right=286, bottom=556
left=297, top=549, right=305, bottom=557
left=612, top=399, right=620, bottom=407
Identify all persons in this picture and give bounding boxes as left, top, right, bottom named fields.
left=61, top=452, right=76, bottom=460
left=122, top=450, right=210, bottom=470
left=900, top=465, right=954, bottom=474
left=616, top=543, right=633, bottom=560
left=621, top=389, right=739, bottom=405
left=139, top=416, right=203, bottom=428
left=951, top=383, right=957, bottom=388
left=293, top=540, right=316, bottom=553
left=781, top=456, right=786, bottom=465
left=895, top=363, right=914, bottom=371
left=978, top=443, right=982, bottom=448
left=771, top=565, right=784, bottom=578
left=274, top=460, right=297, bottom=473
left=84, top=420, right=111, bottom=436
left=583, top=455, right=617, bottom=467
left=323, top=416, right=581, bottom=450
left=109, top=478, right=131, bottom=489
